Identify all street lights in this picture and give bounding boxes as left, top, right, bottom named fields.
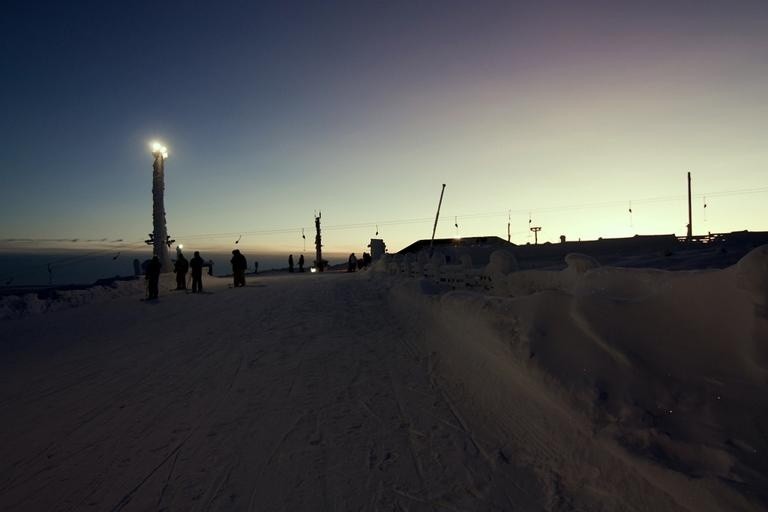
left=148, top=141, right=175, bottom=272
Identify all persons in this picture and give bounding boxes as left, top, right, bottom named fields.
left=298, top=255, right=305, bottom=273
left=346, top=254, right=352, bottom=273
left=236, top=249, right=248, bottom=284
left=173, top=253, right=189, bottom=290
left=144, top=255, right=162, bottom=301
left=288, top=254, right=295, bottom=272
left=230, top=250, right=245, bottom=287
left=189, top=251, right=205, bottom=294
left=351, top=253, right=357, bottom=272
